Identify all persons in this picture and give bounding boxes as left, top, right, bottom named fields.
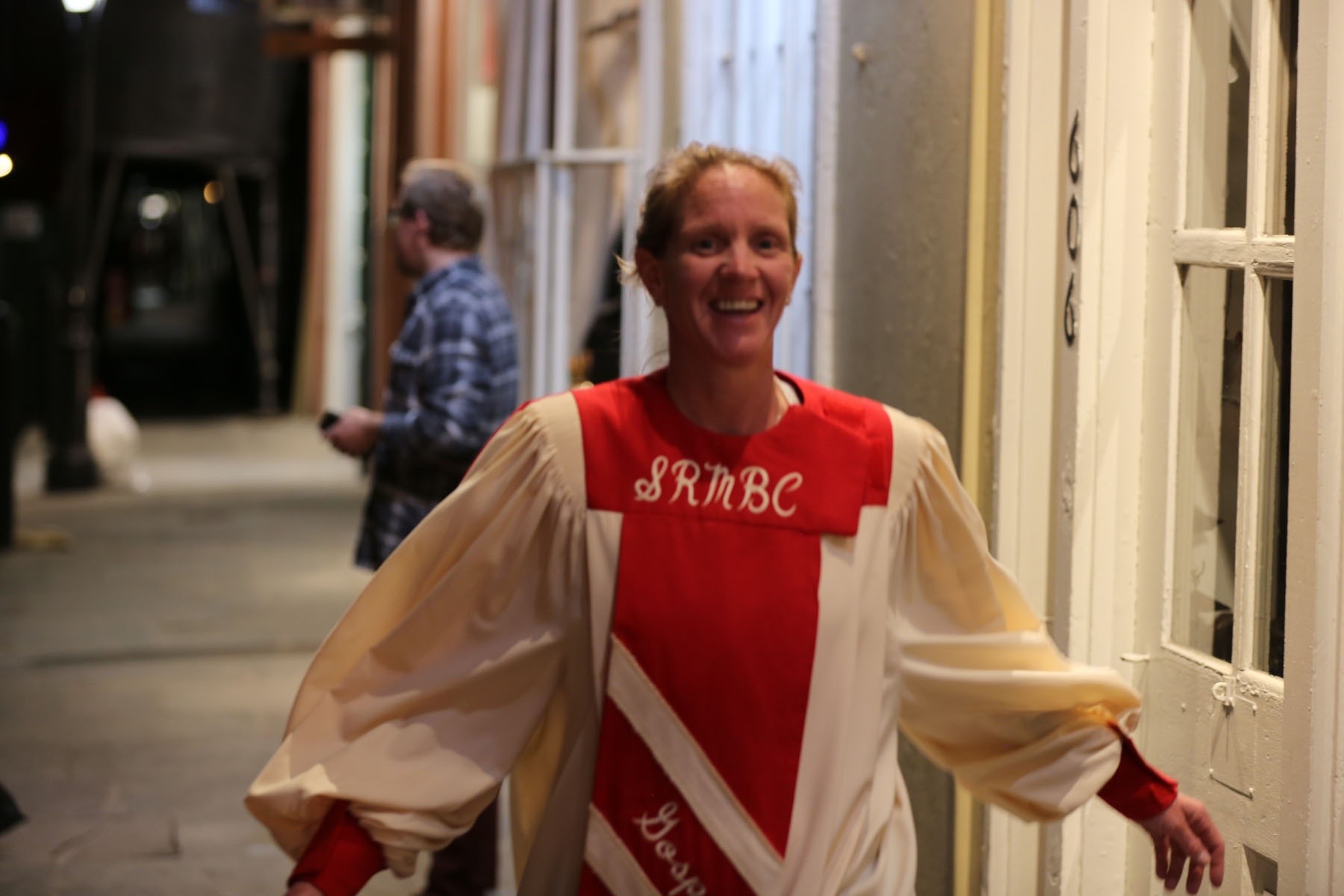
left=549, top=220, right=632, bottom=385
left=263, top=140, right=1239, bottom=896
left=314, top=162, right=522, bottom=896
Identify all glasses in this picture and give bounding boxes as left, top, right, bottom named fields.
left=386, top=195, right=422, bottom=220
